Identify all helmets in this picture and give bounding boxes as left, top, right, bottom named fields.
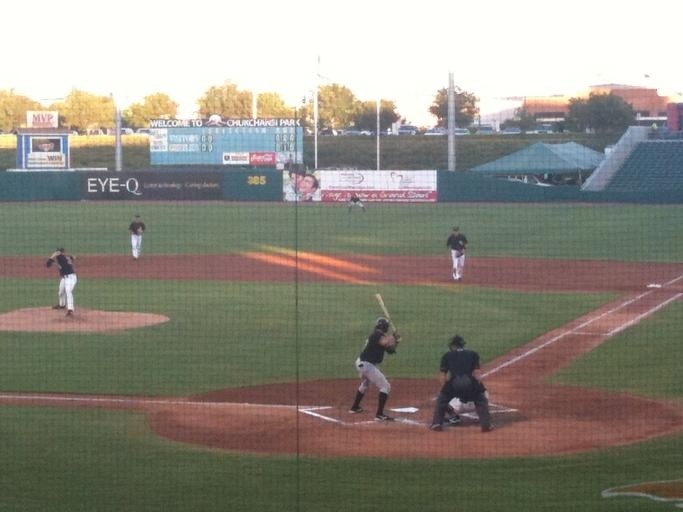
left=376, top=318, right=389, bottom=332
left=447, top=335, right=466, bottom=350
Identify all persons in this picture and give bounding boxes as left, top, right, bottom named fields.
left=446, top=227, right=467, bottom=280
left=299, top=175, right=321, bottom=202
left=285, top=168, right=306, bottom=201
left=128, top=215, right=145, bottom=259
left=46, top=248, right=77, bottom=317
left=429, top=334, right=497, bottom=432
left=349, top=317, right=400, bottom=420
left=348, top=192, right=366, bottom=212
left=444, top=381, right=488, bottom=424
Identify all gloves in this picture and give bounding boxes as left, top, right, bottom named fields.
left=47, top=258, right=54, bottom=267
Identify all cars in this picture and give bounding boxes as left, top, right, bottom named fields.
left=70, top=126, right=149, bottom=136
left=318, top=124, right=559, bottom=136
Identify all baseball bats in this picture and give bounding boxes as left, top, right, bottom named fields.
left=376, top=293, right=402, bottom=342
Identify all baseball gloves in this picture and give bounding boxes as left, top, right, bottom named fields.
left=455, top=249, right=463, bottom=257
left=47, top=259, right=54, bottom=267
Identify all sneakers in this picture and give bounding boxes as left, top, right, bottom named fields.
left=67, top=310, right=72, bottom=315
left=348, top=407, right=362, bottom=414
left=448, top=415, right=460, bottom=424
left=376, top=412, right=389, bottom=421
left=430, top=423, right=440, bottom=430
left=483, top=423, right=496, bottom=430
left=52, top=305, right=65, bottom=309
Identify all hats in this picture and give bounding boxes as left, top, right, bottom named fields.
left=56, top=247, right=64, bottom=252
